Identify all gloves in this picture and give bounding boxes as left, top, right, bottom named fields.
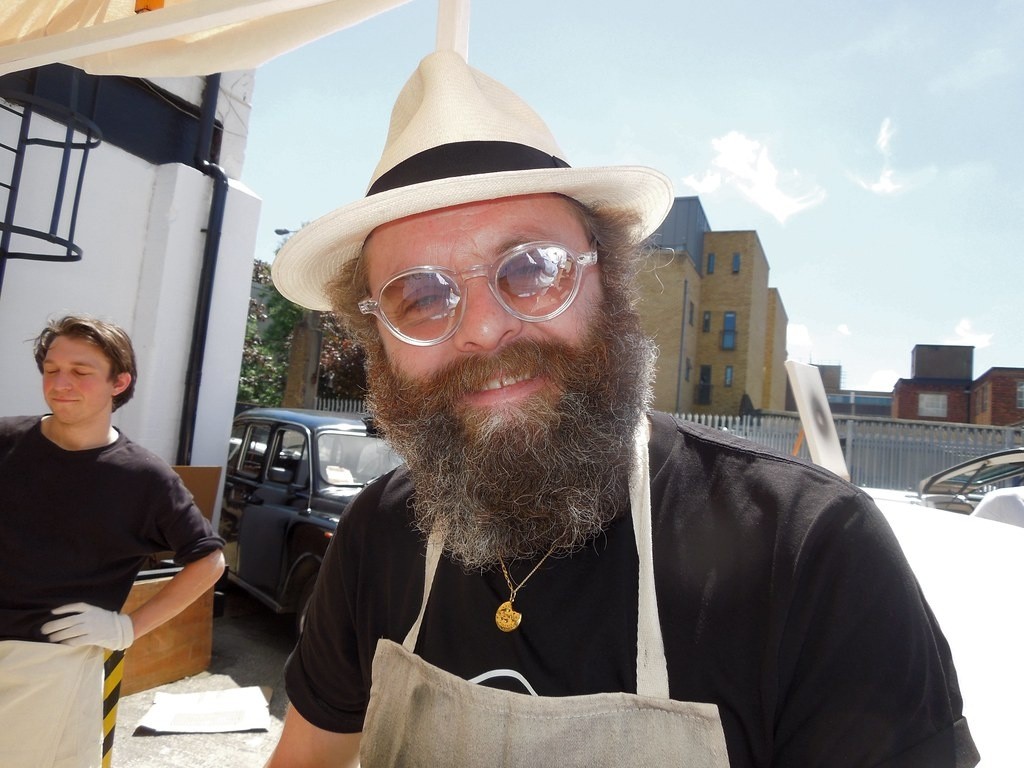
left=41, top=602, right=134, bottom=651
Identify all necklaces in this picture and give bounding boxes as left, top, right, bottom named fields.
left=491, top=539, right=557, bottom=634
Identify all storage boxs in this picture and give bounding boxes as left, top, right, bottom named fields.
left=121, top=467, right=223, bottom=698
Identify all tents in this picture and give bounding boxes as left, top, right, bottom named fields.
left=0, top=0, right=471, bottom=77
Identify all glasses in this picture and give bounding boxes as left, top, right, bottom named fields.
left=357, top=240, right=597, bottom=344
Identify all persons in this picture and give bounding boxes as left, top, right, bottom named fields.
left=1, top=312, right=227, bottom=767
left=252, top=55, right=980, bottom=767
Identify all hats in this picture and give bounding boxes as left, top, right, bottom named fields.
left=269, top=55, right=674, bottom=310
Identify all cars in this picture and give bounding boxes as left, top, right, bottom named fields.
left=217, top=407, right=411, bottom=631
